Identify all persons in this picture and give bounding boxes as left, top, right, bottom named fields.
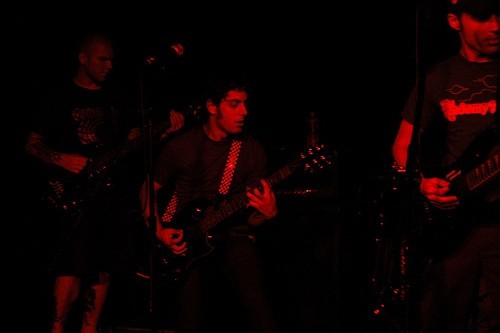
left=139, top=75, right=279, bottom=333
left=25, top=34, right=185, bottom=333
left=392, top=0, right=500, bottom=333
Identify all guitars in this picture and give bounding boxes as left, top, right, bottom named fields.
left=149, top=138, right=331, bottom=272
left=360, top=138, right=498, bottom=275
left=30, top=98, right=202, bottom=210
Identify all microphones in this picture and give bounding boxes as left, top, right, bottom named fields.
left=145, top=44, right=184, bottom=64
left=307, top=112, right=319, bottom=148
